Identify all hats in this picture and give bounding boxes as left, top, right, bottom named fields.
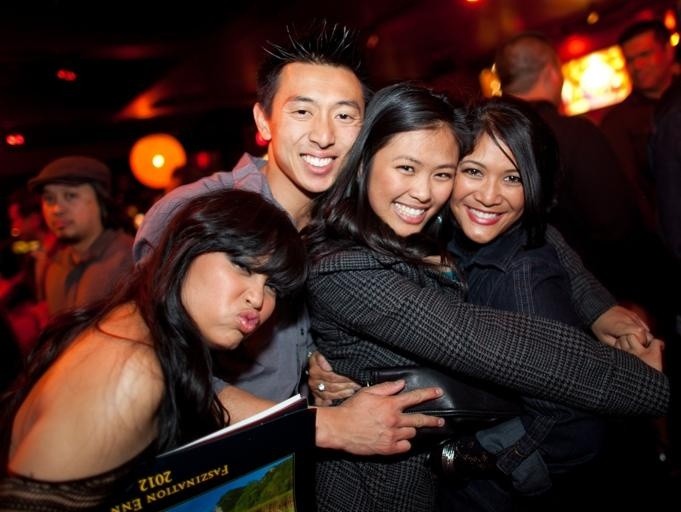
left=32, top=155, right=111, bottom=183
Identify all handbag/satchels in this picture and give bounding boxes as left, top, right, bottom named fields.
left=372, top=364, right=519, bottom=437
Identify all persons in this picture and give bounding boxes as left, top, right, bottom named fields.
left=307, top=94, right=599, bottom=512
left=499, top=20, right=681, bottom=304
left=131, top=23, right=445, bottom=456
left=298, top=83, right=670, bottom=512
left=0, top=156, right=135, bottom=362
left=0, top=187, right=311, bottom=512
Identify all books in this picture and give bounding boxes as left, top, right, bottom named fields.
left=107, top=394, right=317, bottom=512
